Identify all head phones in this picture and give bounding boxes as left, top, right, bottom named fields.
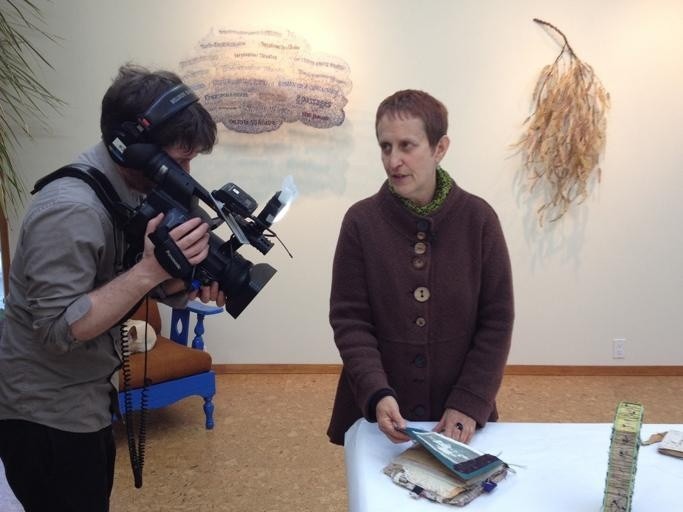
left=107, top=83, right=200, bottom=167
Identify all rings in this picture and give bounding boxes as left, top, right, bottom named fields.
left=456, top=423, right=463, bottom=431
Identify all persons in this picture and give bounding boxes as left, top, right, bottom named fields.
left=0, top=65, right=226, bottom=512
left=327, top=89, right=514, bottom=444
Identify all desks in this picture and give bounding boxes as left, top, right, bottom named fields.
left=345, top=416, right=681, bottom=511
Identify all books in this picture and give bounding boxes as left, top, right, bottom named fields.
left=658, top=431, right=683, bottom=458
left=383, top=428, right=508, bottom=506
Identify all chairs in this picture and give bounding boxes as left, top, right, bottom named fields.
left=112, top=298, right=224, bottom=432
